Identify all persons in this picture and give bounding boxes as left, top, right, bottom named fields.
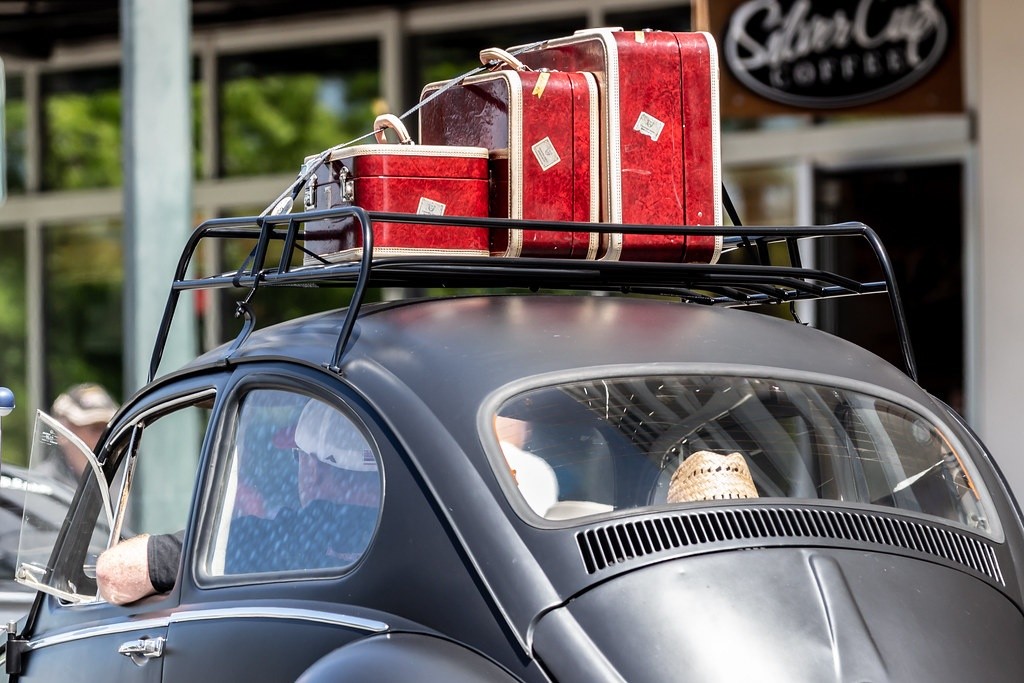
left=96, top=397, right=380, bottom=607
left=28, top=382, right=136, bottom=538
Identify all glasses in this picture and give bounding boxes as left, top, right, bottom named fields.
left=291, top=447, right=311, bottom=462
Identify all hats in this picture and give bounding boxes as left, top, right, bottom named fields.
left=294, top=396, right=381, bottom=472
left=52, top=383, right=120, bottom=427
left=666, top=451, right=758, bottom=503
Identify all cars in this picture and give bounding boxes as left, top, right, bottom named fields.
left=0, top=385, right=163, bottom=654
left=0, top=201, right=1024, bottom=683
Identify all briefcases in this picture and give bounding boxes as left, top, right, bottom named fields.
left=302, top=113, right=489, bottom=266
left=499, top=28, right=723, bottom=265
left=418, top=47, right=600, bottom=261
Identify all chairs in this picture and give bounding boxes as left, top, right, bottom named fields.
left=647, top=414, right=820, bottom=504
